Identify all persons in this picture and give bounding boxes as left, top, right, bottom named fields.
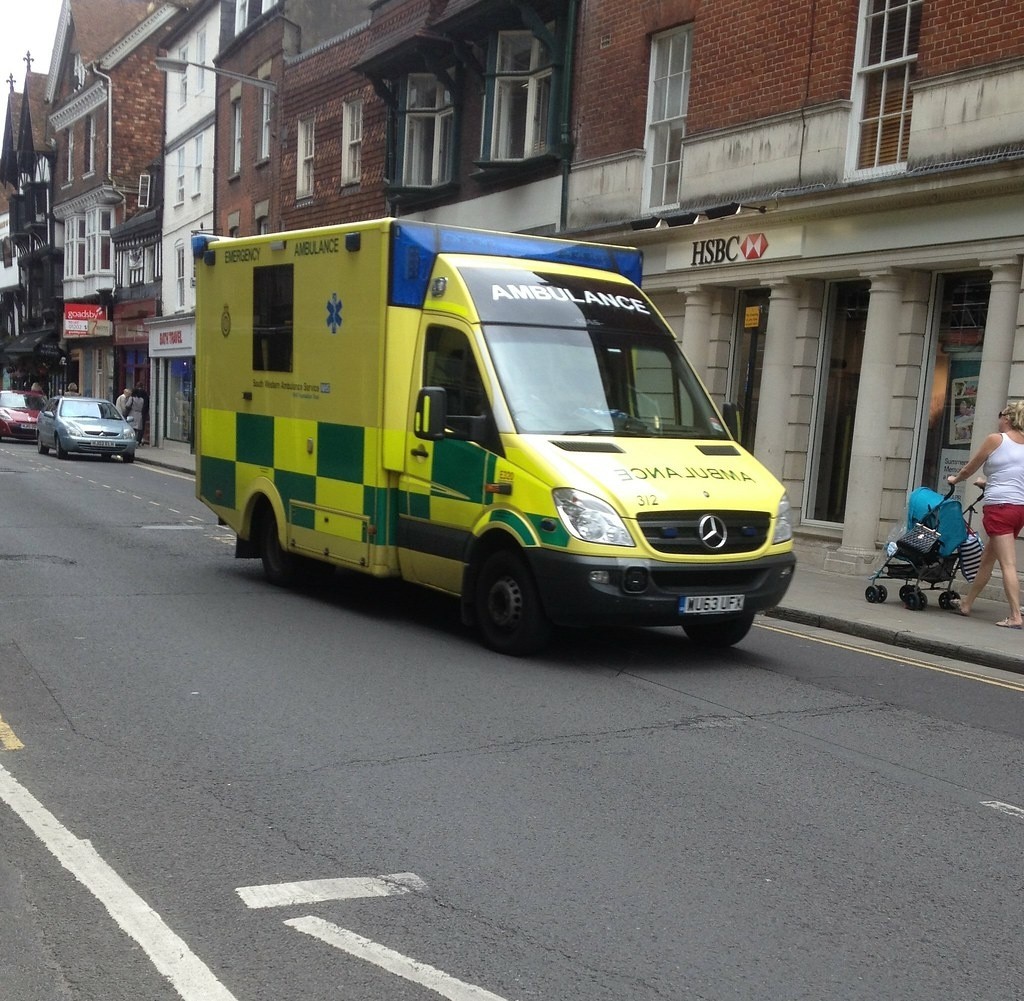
left=949, top=400, right=1024, bottom=628
left=115, top=381, right=149, bottom=447
left=65, top=383, right=79, bottom=396
left=173, top=391, right=190, bottom=440
left=30, top=383, right=47, bottom=401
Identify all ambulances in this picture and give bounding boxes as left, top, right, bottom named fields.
left=191, top=217, right=797, bottom=662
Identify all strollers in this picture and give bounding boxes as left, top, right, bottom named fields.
left=865, top=483, right=984, bottom=611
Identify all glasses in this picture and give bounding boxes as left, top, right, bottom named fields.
left=998, top=411, right=1011, bottom=418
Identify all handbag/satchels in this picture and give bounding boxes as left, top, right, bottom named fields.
left=958, top=520, right=992, bottom=584
left=123, top=398, right=133, bottom=419
left=896, top=507, right=941, bottom=557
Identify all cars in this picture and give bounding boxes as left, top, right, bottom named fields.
left=36, top=396, right=136, bottom=464
left=0, top=390, right=47, bottom=443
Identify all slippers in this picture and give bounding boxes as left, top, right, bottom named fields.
left=950, top=599, right=969, bottom=616
left=996, top=617, right=1022, bottom=628
